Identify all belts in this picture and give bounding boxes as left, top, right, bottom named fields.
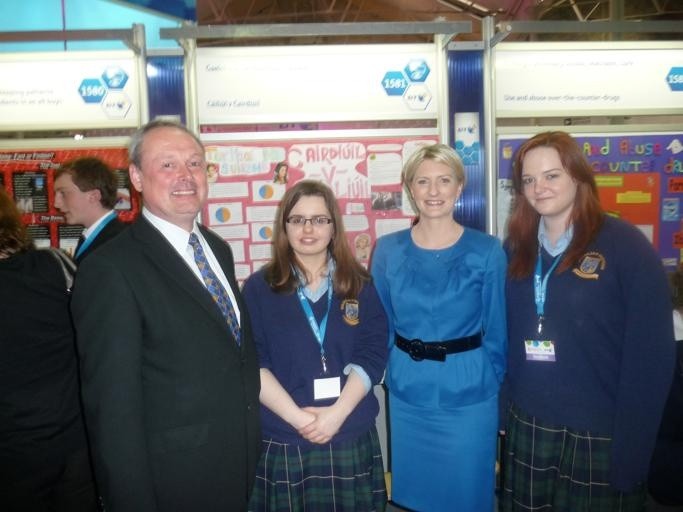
left=394, top=330, right=482, bottom=361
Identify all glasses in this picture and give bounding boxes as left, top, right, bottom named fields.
left=287, top=214, right=335, bottom=225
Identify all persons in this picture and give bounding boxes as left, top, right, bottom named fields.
left=0, top=187, right=102, bottom=512
left=69, top=120, right=260, bottom=511
left=506, top=130, right=675, bottom=511
left=272, top=162, right=288, bottom=185
left=52, top=156, right=133, bottom=266
left=206, top=164, right=217, bottom=181
left=366, top=143, right=510, bottom=512
left=237, top=180, right=391, bottom=511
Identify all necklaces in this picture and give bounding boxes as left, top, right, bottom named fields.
left=417, top=221, right=455, bottom=258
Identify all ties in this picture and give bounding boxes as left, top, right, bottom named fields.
left=188, top=232, right=241, bottom=348
left=74, top=235, right=85, bottom=256
